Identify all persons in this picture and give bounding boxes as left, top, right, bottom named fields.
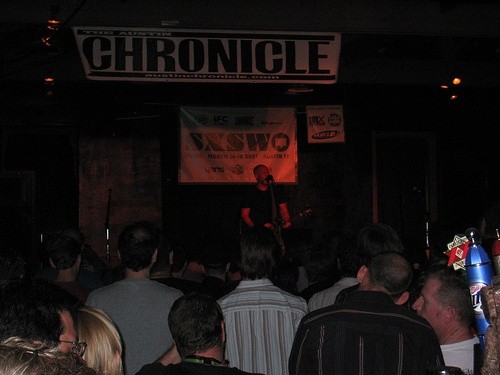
left=241, top=164, right=291, bottom=256
left=0, top=206, right=500, bottom=375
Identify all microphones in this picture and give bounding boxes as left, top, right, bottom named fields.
left=266, top=174, right=274, bottom=184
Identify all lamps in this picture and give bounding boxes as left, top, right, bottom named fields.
left=41, top=4, right=60, bottom=50
